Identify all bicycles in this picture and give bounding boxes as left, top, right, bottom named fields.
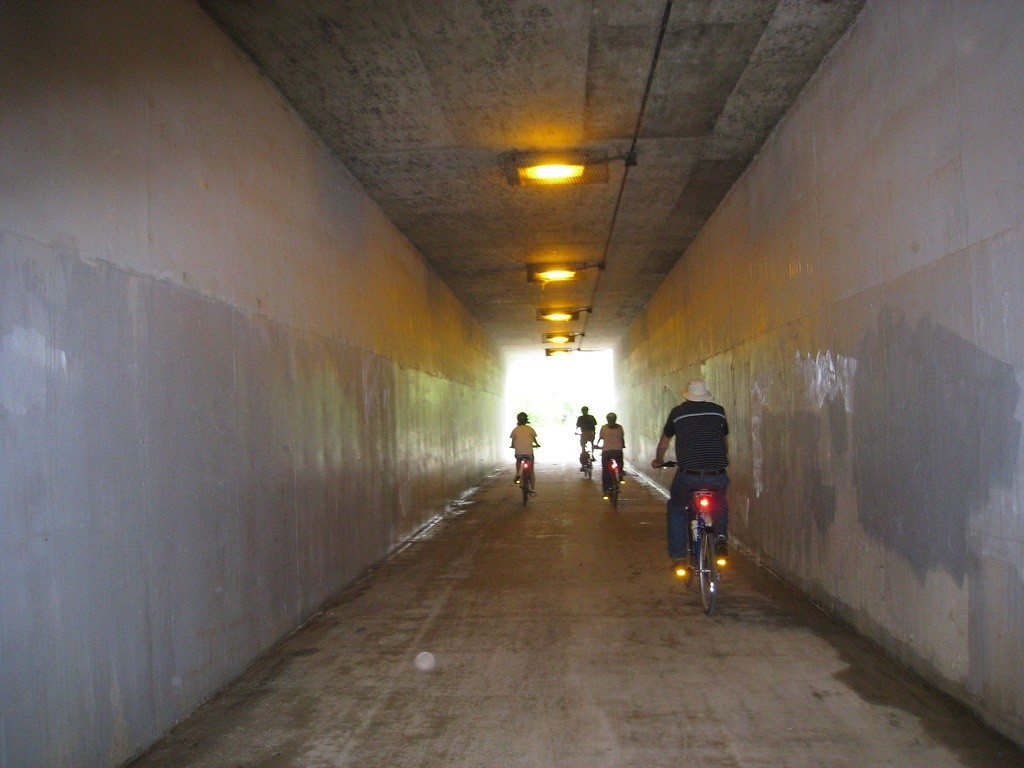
left=655, top=460, right=724, bottom=617
left=593, top=445, right=625, bottom=508
left=576, top=432, right=596, bottom=480
left=509, top=443, right=544, bottom=508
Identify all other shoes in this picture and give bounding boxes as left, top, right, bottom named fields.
left=619, top=471, right=623, bottom=480
left=580, top=467, right=584, bottom=471
left=674, top=558, right=689, bottom=570
left=530, top=490, right=536, bottom=495
left=591, top=456, right=595, bottom=462
left=514, top=475, right=520, bottom=481
left=604, top=490, right=609, bottom=496
left=714, top=535, right=728, bottom=560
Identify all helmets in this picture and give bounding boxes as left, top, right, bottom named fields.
left=517, top=412, right=528, bottom=420
left=582, top=406, right=589, bottom=412
left=606, top=412, right=617, bottom=422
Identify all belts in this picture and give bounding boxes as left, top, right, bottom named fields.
left=686, top=469, right=725, bottom=476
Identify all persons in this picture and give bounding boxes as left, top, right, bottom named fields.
left=596, top=411, right=626, bottom=498
left=510, top=412, right=540, bottom=497
left=575, top=406, right=597, bottom=471
left=651, top=379, right=731, bottom=572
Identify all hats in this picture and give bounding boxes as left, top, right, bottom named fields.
left=680, top=379, right=712, bottom=401
